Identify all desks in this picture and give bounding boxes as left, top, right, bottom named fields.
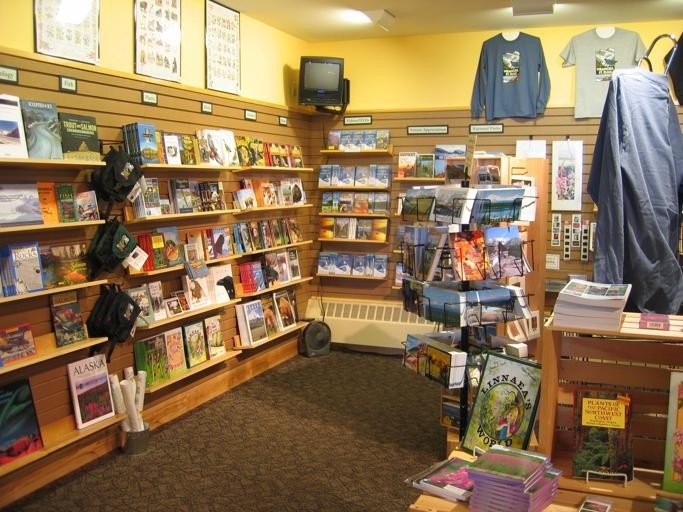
left=408, top=475, right=683, bottom=512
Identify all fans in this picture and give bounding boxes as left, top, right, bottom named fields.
left=302, top=277, right=330, bottom=357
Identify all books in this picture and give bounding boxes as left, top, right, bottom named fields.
left=67, top=354, right=115, bottom=430
left=406, top=443, right=562, bottom=512
left=317, top=129, right=392, bottom=276
left=397, top=143, right=507, bottom=183
left=400, top=187, right=541, bottom=389
left=0, top=93, right=104, bottom=296
left=0, top=379, right=44, bottom=464
left=121, top=123, right=240, bottom=387
left=552, top=279, right=633, bottom=332
left=51, top=291, right=88, bottom=347
left=0, top=323, right=37, bottom=368
left=235, top=135, right=308, bottom=346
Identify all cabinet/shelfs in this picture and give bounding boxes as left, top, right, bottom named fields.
left=391, top=176, right=447, bottom=293
left=129, top=74, right=244, bottom=437
left=318, top=108, right=395, bottom=300
left=232, top=77, right=317, bottom=403
left=1, top=49, right=129, bottom=512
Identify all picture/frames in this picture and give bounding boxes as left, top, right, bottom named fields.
left=33, top=0, right=102, bottom=66
left=133, top=0, right=183, bottom=84
left=204, top=0, right=242, bottom=96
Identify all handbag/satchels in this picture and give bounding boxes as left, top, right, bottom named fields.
left=86, top=145, right=144, bottom=352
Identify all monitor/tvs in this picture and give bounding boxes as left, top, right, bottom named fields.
left=298, top=56, right=344, bottom=107
